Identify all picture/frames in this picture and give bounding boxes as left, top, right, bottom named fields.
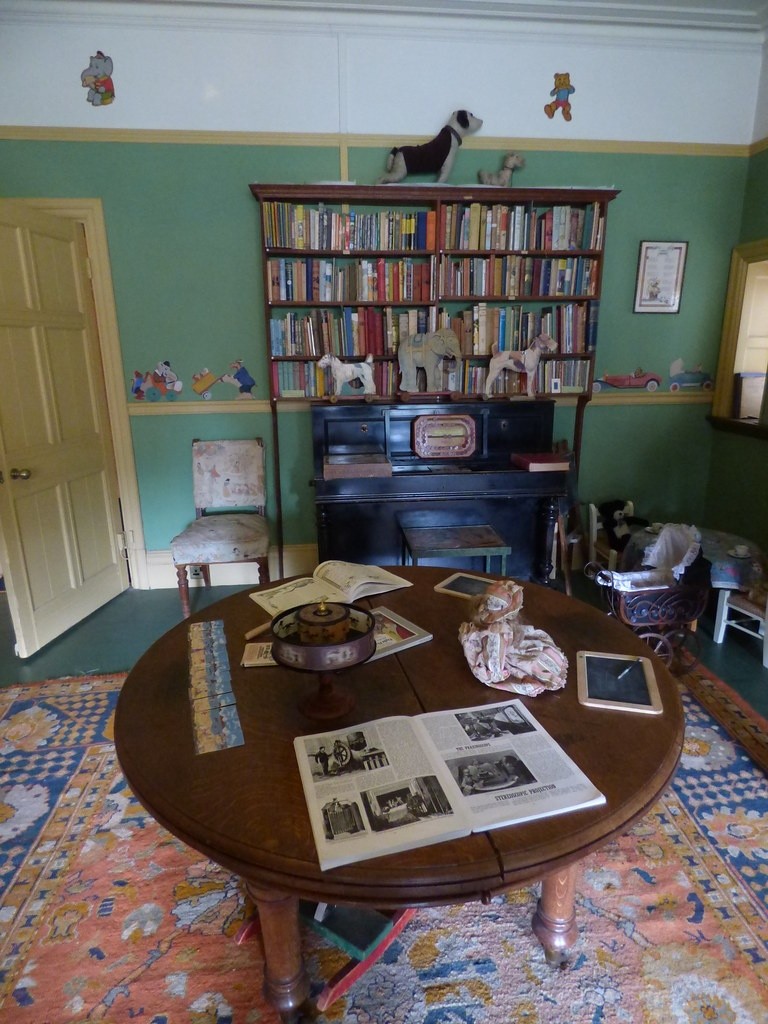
left=633, top=240, right=689, bottom=313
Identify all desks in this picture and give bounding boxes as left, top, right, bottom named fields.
left=117, top=563, right=686, bottom=1023
left=621, top=520, right=768, bottom=644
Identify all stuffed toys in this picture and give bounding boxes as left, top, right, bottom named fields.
left=598, top=500, right=649, bottom=553
left=457, top=580, right=569, bottom=697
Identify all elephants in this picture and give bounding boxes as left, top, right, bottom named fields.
left=398, top=327, right=462, bottom=392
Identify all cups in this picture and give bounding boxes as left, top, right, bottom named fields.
left=735, top=545, right=749, bottom=555
left=652, top=523, right=664, bottom=532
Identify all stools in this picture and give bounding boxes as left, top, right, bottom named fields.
left=403, top=525, right=513, bottom=576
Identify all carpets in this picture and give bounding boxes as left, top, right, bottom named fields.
left=0, top=678, right=768, bottom=1023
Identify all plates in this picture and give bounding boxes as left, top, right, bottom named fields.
left=645, top=527, right=658, bottom=534
left=728, top=549, right=752, bottom=557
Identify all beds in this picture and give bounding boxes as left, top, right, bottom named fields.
left=596, top=523, right=711, bottom=626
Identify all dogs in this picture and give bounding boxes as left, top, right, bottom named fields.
left=484, top=334, right=558, bottom=397
left=377, top=110, right=483, bottom=184
left=318, top=353, right=376, bottom=395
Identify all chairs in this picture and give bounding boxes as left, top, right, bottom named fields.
left=713, top=587, right=768, bottom=669
left=171, top=437, right=271, bottom=623
left=585, top=500, right=636, bottom=572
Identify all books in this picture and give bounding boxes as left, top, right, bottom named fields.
left=266, top=254, right=435, bottom=302
left=363, top=605, right=434, bottom=664
left=269, top=305, right=435, bottom=357
left=442, top=359, right=591, bottom=398
left=263, top=202, right=435, bottom=251
left=440, top=202, right=604, bottom=251
left=248, top=560, right=414, bottom=618
left=293, top=697, right=607, bottom=870
left=271, top=360, right=427, bottom=396
left=734, top=372, right=767, bottom=419
left=439, top=254, right=598, bottom=296
left=438, top=300, right=599, bottom=355
left=511, top=452, right=572, bottom=472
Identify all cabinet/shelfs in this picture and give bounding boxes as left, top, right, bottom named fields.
left=250, top=182, right=624, bottom=579
